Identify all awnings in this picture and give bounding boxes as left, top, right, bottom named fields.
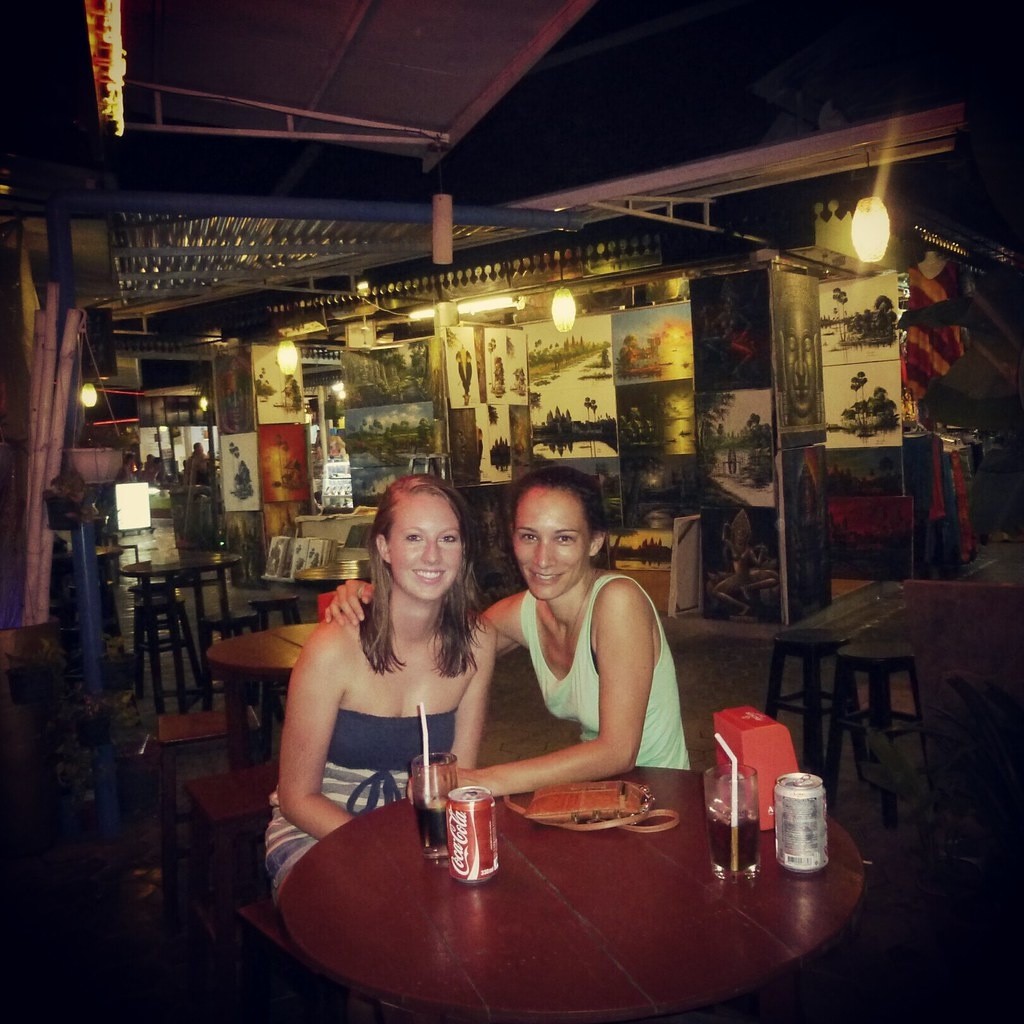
left=108, top=0, right=596, bottom=173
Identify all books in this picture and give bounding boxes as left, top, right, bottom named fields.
left=265, top=535, right=336, bottom=578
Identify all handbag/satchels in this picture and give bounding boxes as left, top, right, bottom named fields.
left=505, top=781, right=681, bottom=832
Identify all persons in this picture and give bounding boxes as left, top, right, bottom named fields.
left=264, top=474, right=498, bottom=904
left=114, top=453, right=134, bottom=482
left=183, top=442, right=210, bottom=484
left=143, top=453, right=165, bottom=481
left=710, top=522, right=778, bottom=616
left=43, top=469, right=111, bottom=522
left=324, top=465, right=691, bottom=804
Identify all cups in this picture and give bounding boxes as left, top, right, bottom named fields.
left=411, top=752, right=458, bottom=858
left=703, top=764, right=760, bottom=881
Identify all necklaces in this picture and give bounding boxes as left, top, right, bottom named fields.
left=570, top=568, right=598, bottom=633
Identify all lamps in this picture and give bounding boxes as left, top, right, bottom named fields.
left=80, top=382, right=99, bottom=408
left=276, top=338, right=299, bottom=377
left=407, top=294, right=520, bottom=318
left=551, top=266, right=581, bottom=335
left=847, top=176, right=890, bottom=264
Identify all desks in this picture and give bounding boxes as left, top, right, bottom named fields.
left=119, top=550, right=243, bottom=614
left=279, top=762, right=867, bottom=1024
left=206, top=622, right=325, bottom=772
left=295, top=558, right=371, bottom=589
left=47, top=546, right=124, bottom=639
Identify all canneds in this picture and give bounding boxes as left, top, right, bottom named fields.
left=774, top=773, right=829, bottom=873
left=445, top=785, right=499, bottom=883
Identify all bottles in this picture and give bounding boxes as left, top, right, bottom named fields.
left=311, top=415, right=353, bottom=509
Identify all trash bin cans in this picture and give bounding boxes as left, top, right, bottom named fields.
left=168, top=486, right=218, bottom=550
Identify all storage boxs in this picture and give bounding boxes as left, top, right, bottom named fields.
left=710, top=700, right=797, bottom=832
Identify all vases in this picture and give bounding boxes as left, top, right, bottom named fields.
left=76, top=710, right=117, bottom=750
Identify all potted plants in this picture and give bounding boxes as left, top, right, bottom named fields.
left=4, top=639, right=68, bottom=709
left=61, top=419, right=139, bottom=487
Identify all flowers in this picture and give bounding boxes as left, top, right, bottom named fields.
left=75, top=692, right=105, bottom=716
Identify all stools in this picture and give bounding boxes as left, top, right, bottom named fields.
left=816, top=643, right=952, bottom=830
left=154, top=711, right=227, bottom=948
left=250, top=595, right=308, bottom=708
left=199, top=608, right=286, bottom=725
left=127, top=582, right=185, bottom=699
left=236, top=895, right=348, bottom=1024
left=133, top=596, right=205, bottom=717
left=766, top=624, right=846, bottom=776
left=183, top=761, right=280, bottom=977
